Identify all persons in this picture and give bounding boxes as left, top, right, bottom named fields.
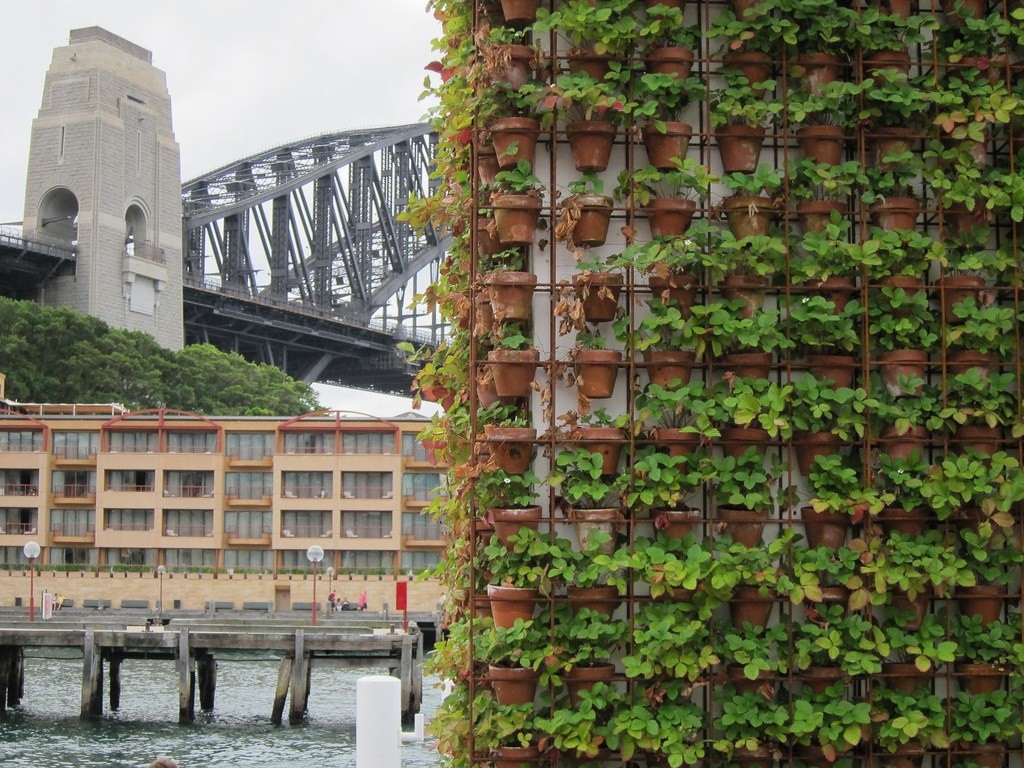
left=358, top=590, right=368, bottom=611
left=440, top=592, right=447, bottom=606
left=329, top=590, right=349, bottom=614
left=52, top=593, right=64, bottom=611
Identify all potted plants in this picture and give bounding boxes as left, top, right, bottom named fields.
left=395, top=0, right=1024, bottom=768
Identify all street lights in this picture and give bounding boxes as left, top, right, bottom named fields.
left=306, top=545, right=324, bottom=626
left=157, top=565, right=166, bottom=614
left=24, top=542, right=41, bottom=622
left=325, top=567, right=335, bottom=596
